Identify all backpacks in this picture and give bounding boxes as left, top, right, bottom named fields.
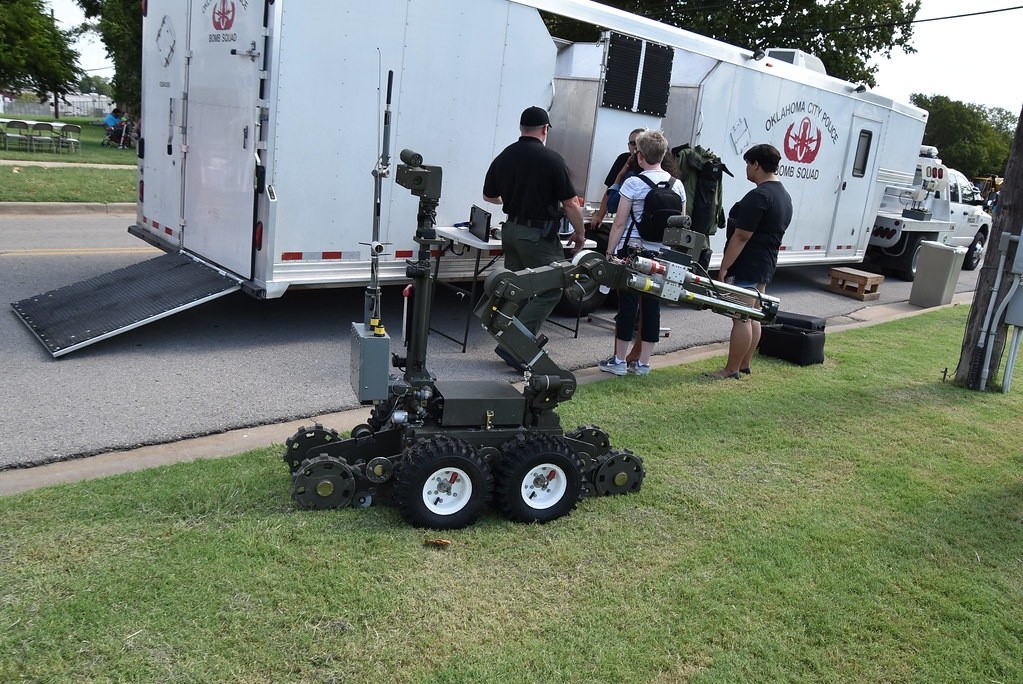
left=630, top=174, right=683, bottom=242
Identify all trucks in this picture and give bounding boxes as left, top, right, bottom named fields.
left=8, top=0, right=996, bottom=363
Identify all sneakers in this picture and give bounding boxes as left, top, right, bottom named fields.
left=629, top=360, right=650, bottom=376
left=600, top=355, right=628, bottom=376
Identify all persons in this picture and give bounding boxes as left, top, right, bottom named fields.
left=599, top=128, right=687, bottom=376
left=983, top=188, right=1002, bottom=225
left=605, top=148, right=686, bottom=374
left=701, top=144, right=793, bottom=379
left=590, top=129, right=645, bottom=230
left=482, top=106, right=587, bottom=374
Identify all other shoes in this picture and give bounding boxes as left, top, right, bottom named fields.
left=495, top=347, right=525, bottom=375
left=740, top=368, right=750, bottom=374
left=702, top=371, right=739, bottom=380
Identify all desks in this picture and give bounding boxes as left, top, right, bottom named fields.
left=0, top=118, right=76, bottom=154
left=427, top=226, right=597, bottom=353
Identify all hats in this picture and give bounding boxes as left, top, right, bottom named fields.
left=520, top=106, right=552, bottom=128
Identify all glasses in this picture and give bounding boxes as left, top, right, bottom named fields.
left=629, top=141, right=636, bottom=146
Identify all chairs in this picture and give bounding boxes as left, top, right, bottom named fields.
left=56, top=124, right=82, bottom=156
left=3, top=120, right=30, bottom=152
left=28, top=123, right=55, bottom=154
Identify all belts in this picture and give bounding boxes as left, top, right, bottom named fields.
left=508, top=215, right=544, bottom=229
